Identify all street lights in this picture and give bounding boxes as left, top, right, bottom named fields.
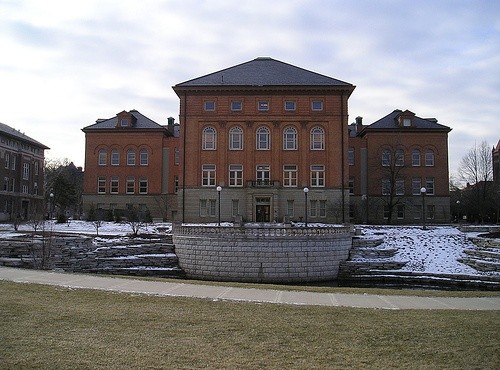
left=421, top=187, right=427, bottom=230
left=49, top=193, right=54, bottom=220
left=216, top=185, right=223, bottom=226
left=304, top=187, right=309, bottom=227
